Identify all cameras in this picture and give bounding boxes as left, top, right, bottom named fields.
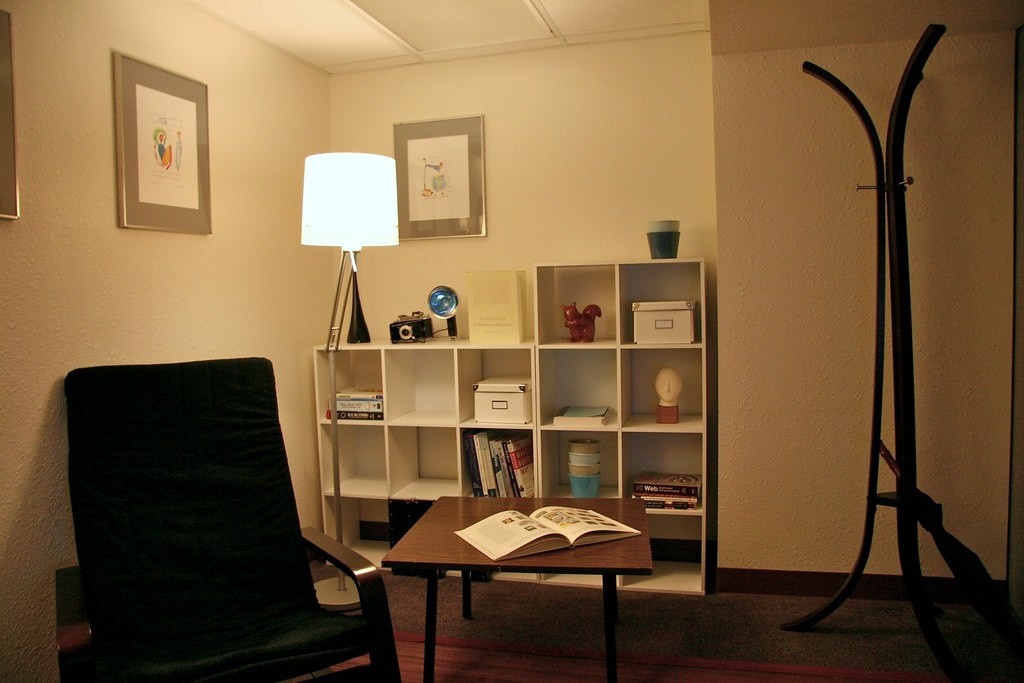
left=390, top=315, right=433, bottom=344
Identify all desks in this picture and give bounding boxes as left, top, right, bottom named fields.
left=381, top=497, right=655, bottom=683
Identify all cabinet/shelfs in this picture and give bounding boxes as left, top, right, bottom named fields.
left=533, top=257, right=707, bottom=596
left=312, top=338, right=539, bottom=585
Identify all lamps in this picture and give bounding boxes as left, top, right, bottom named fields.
left=427, top=286, right=459, bottom=342
left=301, top=152, right=401, bottom=612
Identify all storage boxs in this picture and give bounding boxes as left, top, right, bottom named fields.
left=472, top=376, right=532, bottom=424
left=631, top=299, right=695, bottom=345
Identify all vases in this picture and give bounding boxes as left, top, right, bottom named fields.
left=647, top=219, right=679, bottom=233
left=567, top=439, right=601, bottom=498
left=647, top=231, right=681, bottom=259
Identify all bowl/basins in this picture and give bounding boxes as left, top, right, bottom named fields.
left=648, top=220, right=680, bottom=231
left=646, top=231, right=681, bottom=259
left=567, top=439, right=601, bottom=498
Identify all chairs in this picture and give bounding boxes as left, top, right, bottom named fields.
left=51, top=356, right=403, bottom=682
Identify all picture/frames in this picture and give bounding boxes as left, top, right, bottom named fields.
left=112, top=51, right=212, bottom=237
left=392, top=114, right=487, bottom=243
left=0, top=8, right=21, bottom=221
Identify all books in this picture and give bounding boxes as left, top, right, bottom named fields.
left=466, top=271, right=526, bottom=344
left=463, top=430, right=533, bottom=498
left=632, top=471, right=702, bottom=510
left=553, top=406, right=610, bottom=426
left=455, top=506, right=641, bottom=562
left=328, top=387, right=383, bottom=420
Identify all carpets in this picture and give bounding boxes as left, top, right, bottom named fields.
left=317, top=562, right=1024, bottom=683
